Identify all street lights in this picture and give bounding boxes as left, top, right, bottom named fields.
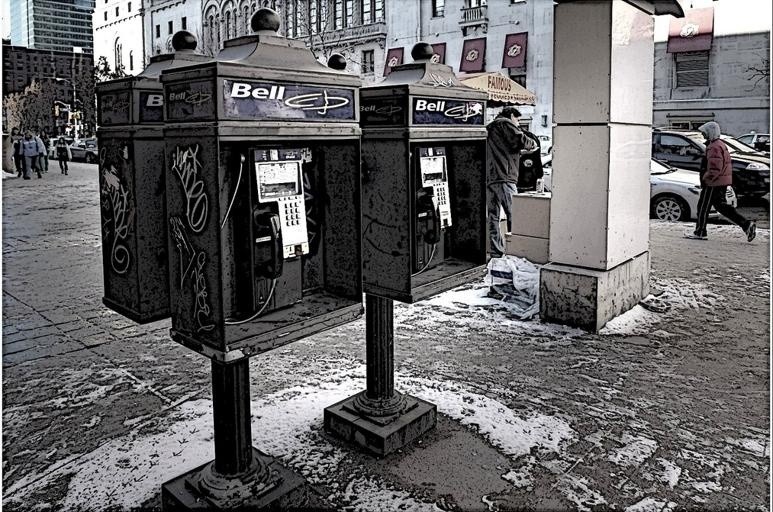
left=55, top=78, right=79, bottom=147
left=54, top=100, right=70, bottom=136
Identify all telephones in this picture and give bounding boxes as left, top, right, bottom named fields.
left=416, top=147, right=454, bottom=243
left=244, top=147, right=310, bottom=279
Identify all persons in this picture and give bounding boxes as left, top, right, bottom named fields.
left=486, top=106, right=536, bottom=261
left=54, top=137, right=73, bottom=176
left=11, top=126, right=50, bottom=180
left=683, top=122, right=756, bottom=241
left=517, top=127, right=544, bottom=193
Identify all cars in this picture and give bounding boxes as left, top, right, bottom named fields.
left=47, top=136, right=98, bottom=162
left=536, top=124, right=770, bottom=223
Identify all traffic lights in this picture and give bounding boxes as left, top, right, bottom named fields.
left=70, top=112, right=79, bottom=118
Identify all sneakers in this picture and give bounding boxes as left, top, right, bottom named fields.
left=746, top=221, right=756, bottom=242
left=61, top=169, right=69, bottom=176
left=683, top=231, right=708, bottom=241
left=18, top=169, right=49, bottom=180
